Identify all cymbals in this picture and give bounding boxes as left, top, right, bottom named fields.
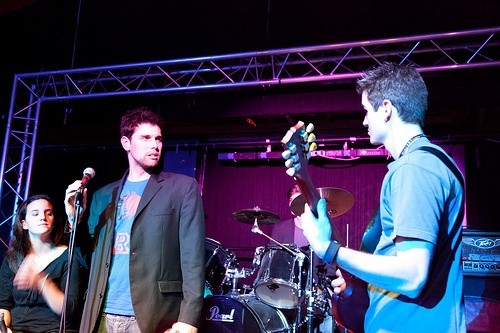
left=289, top=186, right=356, bottom=219
left=232, top=209, right=279, bottom=225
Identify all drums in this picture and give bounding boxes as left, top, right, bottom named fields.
left=252, top=243, right=310, bottom=310
left=199, top=293, right=291, bottom=333
left=202, top=238, right=239, bottom=299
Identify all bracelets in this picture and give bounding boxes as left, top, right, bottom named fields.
left=38, top=273, right=50, bottom=295
left=68, top=224, right=72, bottom=229
left=324, top=240, right=342, bottom=265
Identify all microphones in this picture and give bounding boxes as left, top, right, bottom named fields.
left=74, top=166, right=96, bottom=198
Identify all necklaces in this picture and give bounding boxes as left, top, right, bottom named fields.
left=399, top=134, right=425, bottom=158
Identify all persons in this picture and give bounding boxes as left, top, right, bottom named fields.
left=300, top=62, right=470, bottom=333
left=65, top=108, right=205, bottom=333
left=268, top=185, right=335, bottom=333
left=0, top=194, right=88, bottom=333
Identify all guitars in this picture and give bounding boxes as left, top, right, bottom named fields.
left=280, top=120, right=370, bottom=333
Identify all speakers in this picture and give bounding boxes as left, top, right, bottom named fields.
left=462, top=275, right=500, bottom=333
